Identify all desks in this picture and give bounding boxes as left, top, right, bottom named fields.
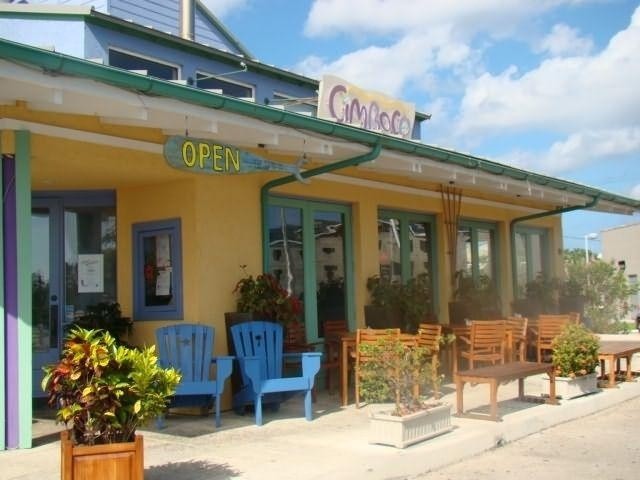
left=455, top=361, right=562, bottom=423
left=594, top=340, right=640, bottom=389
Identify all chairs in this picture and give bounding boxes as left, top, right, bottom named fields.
left=229, top=320, right=324, bottom=426
left=154, top=323, right=234, bottom=431
left=281, top=312, right=581, bottom=410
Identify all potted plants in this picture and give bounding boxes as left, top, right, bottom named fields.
left=542, top=323, right=598, bottom=401
left=358, top=329, right=457, bottom=447
left=39, top=326, right=180, bottom=480
left=224, top=263, right=304, bottom=417
left=369, top=270, right=597, bottom=369
